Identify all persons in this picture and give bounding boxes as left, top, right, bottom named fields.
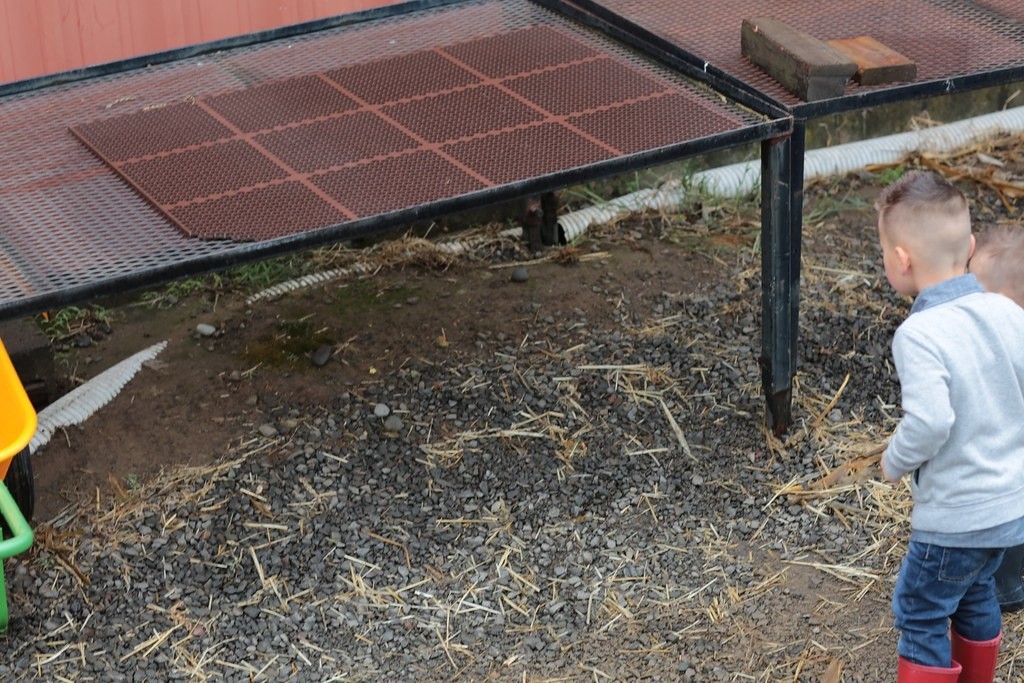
left=968, top=223, right=1023, bottom=614
left=876, top=165, right=1024, bottom=683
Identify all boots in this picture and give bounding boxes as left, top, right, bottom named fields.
left=898, top=622, right=1003, bottom=683
